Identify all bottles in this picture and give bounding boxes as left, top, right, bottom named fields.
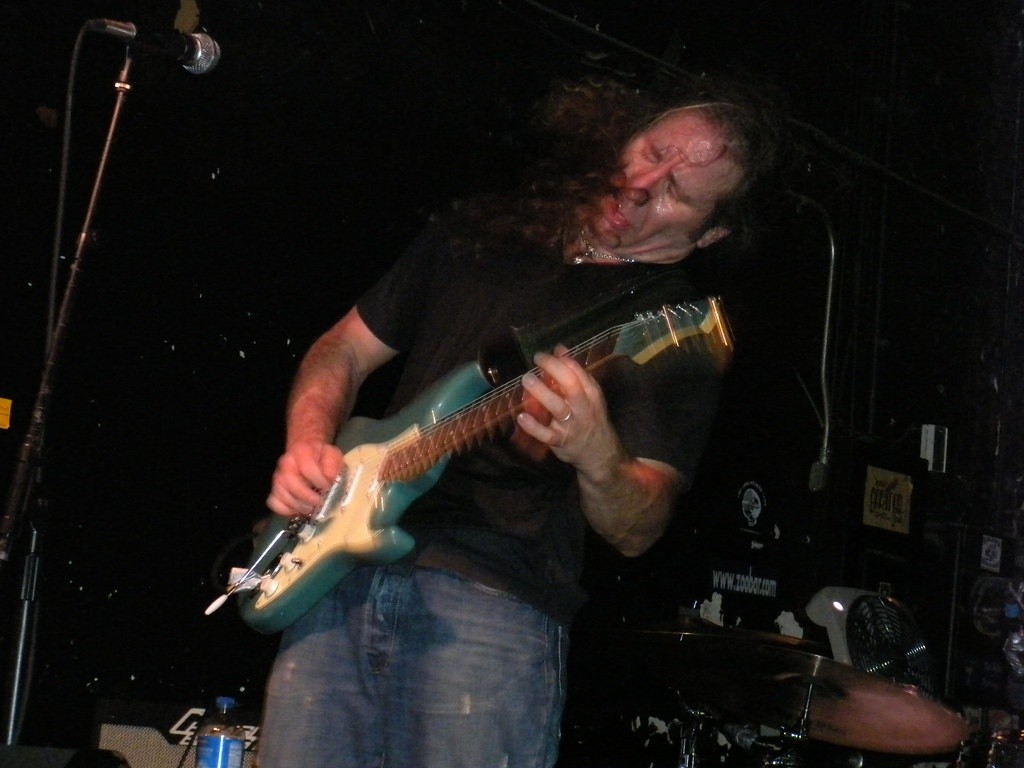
left=195, top=696, right=246, bottom=768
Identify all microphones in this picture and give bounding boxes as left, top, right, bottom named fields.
left=90, top=19, right=222, bottom=73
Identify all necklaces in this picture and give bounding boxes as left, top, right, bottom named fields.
left=570, top=229, right=635, bottom=266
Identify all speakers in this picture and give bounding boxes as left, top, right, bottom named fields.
left=91, top=699, right=263, bottom=768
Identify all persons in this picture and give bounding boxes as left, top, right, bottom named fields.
left=254, top=76, right=771, bottom=768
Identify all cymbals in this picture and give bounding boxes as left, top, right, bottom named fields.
left=695, top=631, right=972, bottom=754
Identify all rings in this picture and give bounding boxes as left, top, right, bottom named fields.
left=559, top=408, right=572, bottom=422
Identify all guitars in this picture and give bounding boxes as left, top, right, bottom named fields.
left=205, top=296, right=736, bottom=637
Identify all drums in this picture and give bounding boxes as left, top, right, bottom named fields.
left=985, top=728, right=1024, bottom=767
